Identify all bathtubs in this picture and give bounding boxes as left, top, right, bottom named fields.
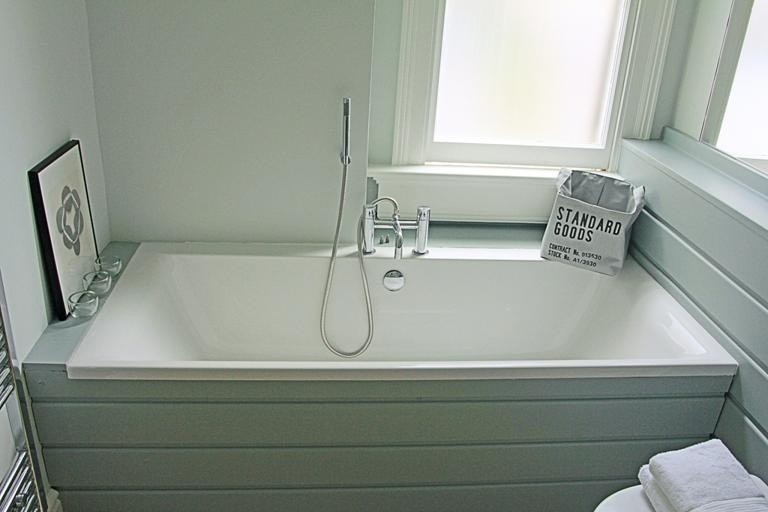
left=63, top=243, right=740, bottom=381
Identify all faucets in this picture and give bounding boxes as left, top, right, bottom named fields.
left=361, top=199, right=431, bottom=258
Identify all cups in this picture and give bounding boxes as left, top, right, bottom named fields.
left=95, top=255, right=121, bottom=280
left=67, top=290, right=99, bottom=321
left=83, top=270, right=112, bottom=298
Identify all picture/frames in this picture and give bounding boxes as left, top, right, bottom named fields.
left=27, top=139, right=102, bottom=321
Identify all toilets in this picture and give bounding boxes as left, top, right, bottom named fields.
left=595, top=473, right=768, bottom=512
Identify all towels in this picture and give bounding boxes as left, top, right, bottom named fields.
left=639, top=437, right=768, bottom=512
